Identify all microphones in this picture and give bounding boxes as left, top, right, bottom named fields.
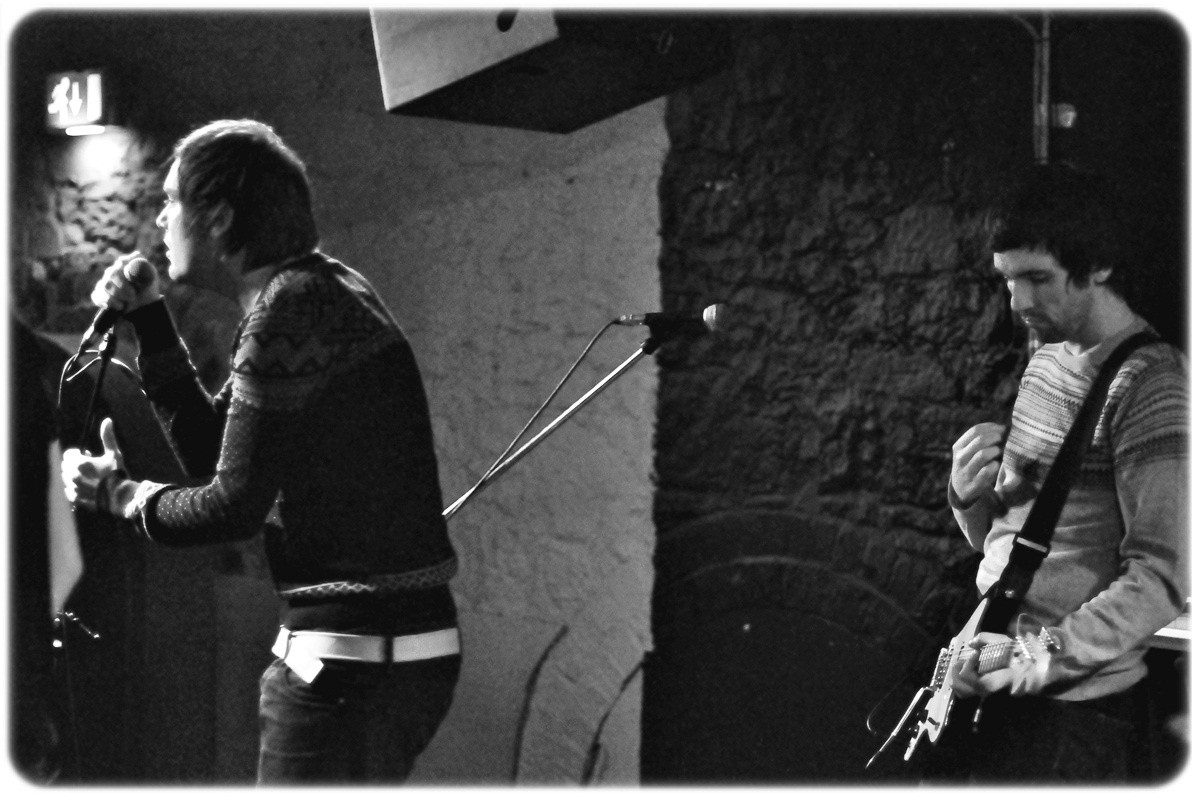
left=79, top=258, right=158, bottom=357
left=612, top=304, right=735, bottom=334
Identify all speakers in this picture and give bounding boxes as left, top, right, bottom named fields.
left=365, top=6, right=734, bottom=134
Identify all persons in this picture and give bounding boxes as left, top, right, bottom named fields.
left=60, top=121, right=463, bottom=784
left=946, top=196, right=1187, bottom=783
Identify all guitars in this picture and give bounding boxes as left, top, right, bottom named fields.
left=904, top=597, right=1064, bottom=764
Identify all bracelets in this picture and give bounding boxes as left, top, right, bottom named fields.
left=948, top=474, right=979, bottom=510
left=96, top=468, right=127, bottom=519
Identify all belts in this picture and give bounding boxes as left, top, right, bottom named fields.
left=272, top=623, right=462, bottom=683
